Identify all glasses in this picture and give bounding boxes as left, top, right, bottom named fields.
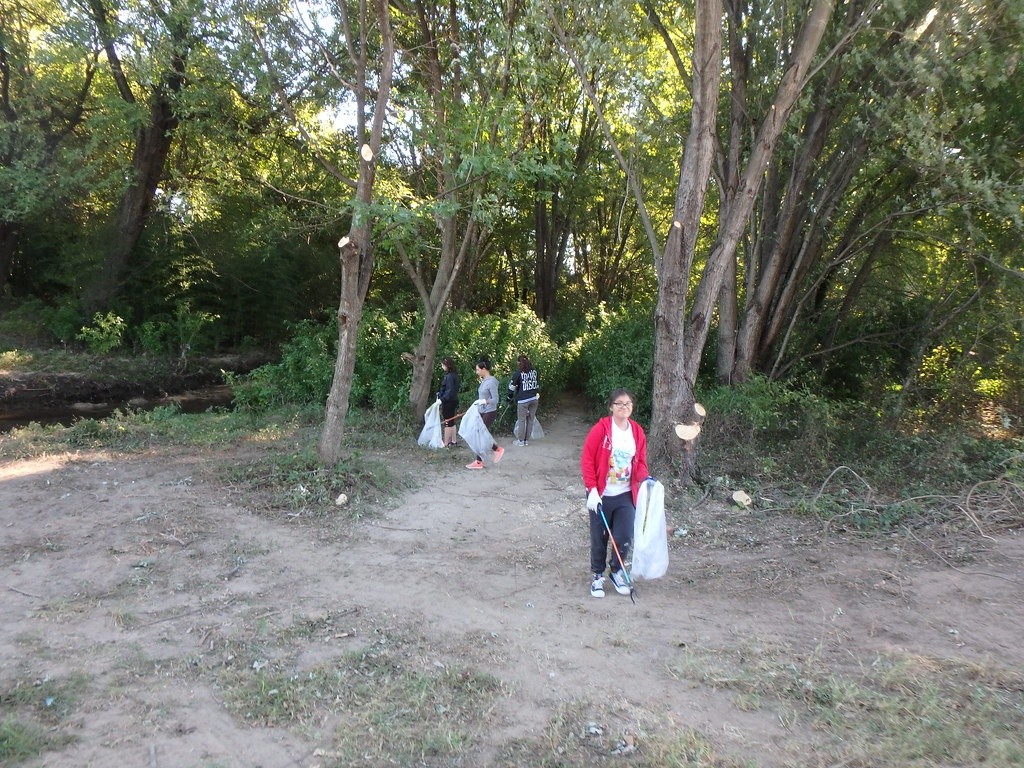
left=612, top=400, right=633, bottom=408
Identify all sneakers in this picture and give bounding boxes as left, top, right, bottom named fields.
left=524, top=440, right=529, bottom=446
left=608, top=569, right=630, bottom=595
left=464, top=461, right=483, bottom=469
left=590, top=575, right=606, bottom=597
left=512, top=439, right=524, bottom=446
left=493, top=447, right=504, bottom=463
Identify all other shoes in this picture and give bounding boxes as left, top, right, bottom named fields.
left=444, top=442, right=457, bottom=449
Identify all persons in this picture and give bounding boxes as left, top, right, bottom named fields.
left=506, top=353, right=540, bottom=446
left=434, top=356, right=459, bottom=450
left=580, top=389, right=657, bottom=598
left=466, top=360, right=504, bottom=469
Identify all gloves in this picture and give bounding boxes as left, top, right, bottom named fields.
left=473, top=399, right=487, bottom=407
left=436, top=399, right=442, bottom=406
left=586, top=488, right=603, bottom=514
